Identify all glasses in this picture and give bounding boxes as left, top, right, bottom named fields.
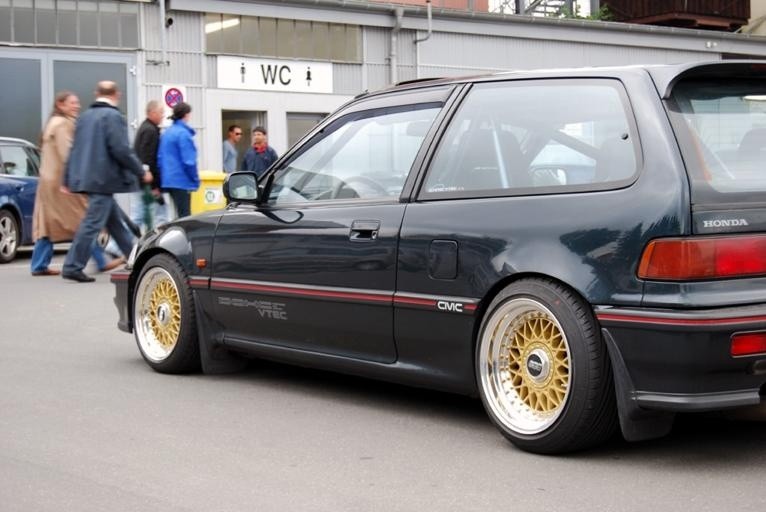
left=235, top=132, right=242, bottom=135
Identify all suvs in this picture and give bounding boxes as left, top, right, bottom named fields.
left=112, top=58, right=766, bottom=457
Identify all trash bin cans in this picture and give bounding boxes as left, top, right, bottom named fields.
left=189, top=171, right=230, bottom=216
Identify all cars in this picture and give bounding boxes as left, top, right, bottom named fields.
left=0, top=135, right=41, bottom=264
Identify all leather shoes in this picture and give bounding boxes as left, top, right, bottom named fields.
left=31, top=269, right=59, bottom=275
left=62, top=271, right=94, bottom=282
left=99, top=256, right=124, bottom=271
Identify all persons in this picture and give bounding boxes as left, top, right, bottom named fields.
left=61, top=80, right=154, bottom=282
left=241, top=126, right=278, bottom=176
left=29, top=91, right=124, bottom=274
left=223, top=124, right=243, bottom=173
left=157, top=102, right=201, bottom=217
left=135, top=100, right=163, bottom=232
